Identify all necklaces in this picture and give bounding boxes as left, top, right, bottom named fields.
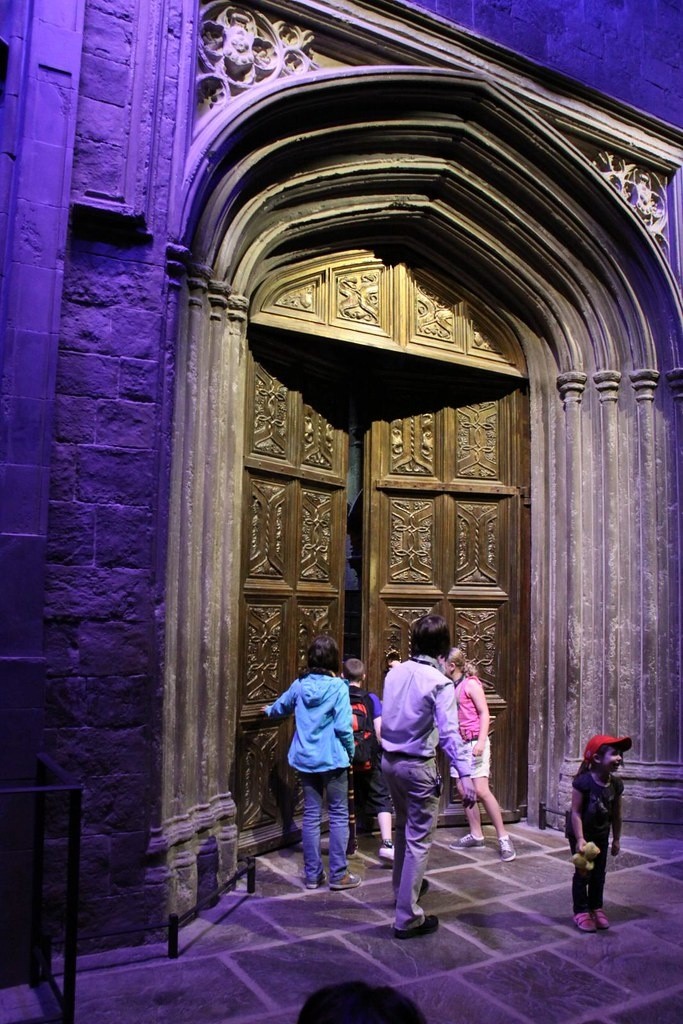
left=411, top=658, right=436, bottom=668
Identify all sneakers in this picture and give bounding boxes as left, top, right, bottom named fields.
left=450, top=833, right=487, bottom=850
left=498, top=834, right=516, bottom=861
left=329, top=873, right=362, bottom=889
left=307, top=871, right=326, bottom=888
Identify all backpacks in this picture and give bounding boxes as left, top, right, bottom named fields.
left=348, top=689, right=378, bottom=771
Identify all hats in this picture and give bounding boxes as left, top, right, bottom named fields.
left=585, top=734, right=632, bottom=763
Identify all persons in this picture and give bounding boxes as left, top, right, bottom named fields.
left=259, top=636, right=362, bottom=888
left=389, top=648, right=516, bottom=862
left=381, top=616, right=476, bottom=940
left=565, top=734, right=632, bottom=931
left=341, top=658, right=394, bottom=860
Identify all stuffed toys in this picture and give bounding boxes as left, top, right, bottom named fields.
left=569, top=842, right=600, bottom=875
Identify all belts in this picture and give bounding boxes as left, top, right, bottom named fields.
left=396, top=752, right=427, bottom=759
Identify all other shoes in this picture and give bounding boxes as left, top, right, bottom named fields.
left=590, top=908, right=609, bottom=928
left=572, top=912, right=600, bottom=932
left=394, top=879, right=429, bottom=907
left=394, top=915, right=438, bottom=939
left=378, top=841, right=394, bottom=863
